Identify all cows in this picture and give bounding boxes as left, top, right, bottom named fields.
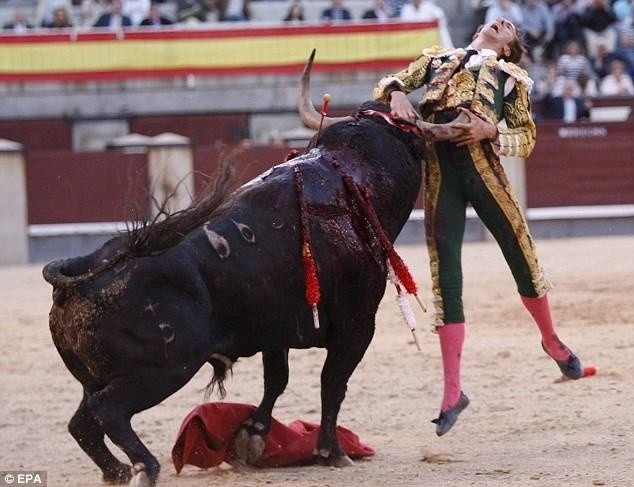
left=42, top=47, right=471, bottom=487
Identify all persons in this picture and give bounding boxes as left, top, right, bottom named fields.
left=371, top=17, right=584, bottom=437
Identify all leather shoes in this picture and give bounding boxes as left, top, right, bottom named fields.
left=432, top=389, right=470, bottom=439
left=538, top=331, right=584, bottom=380
